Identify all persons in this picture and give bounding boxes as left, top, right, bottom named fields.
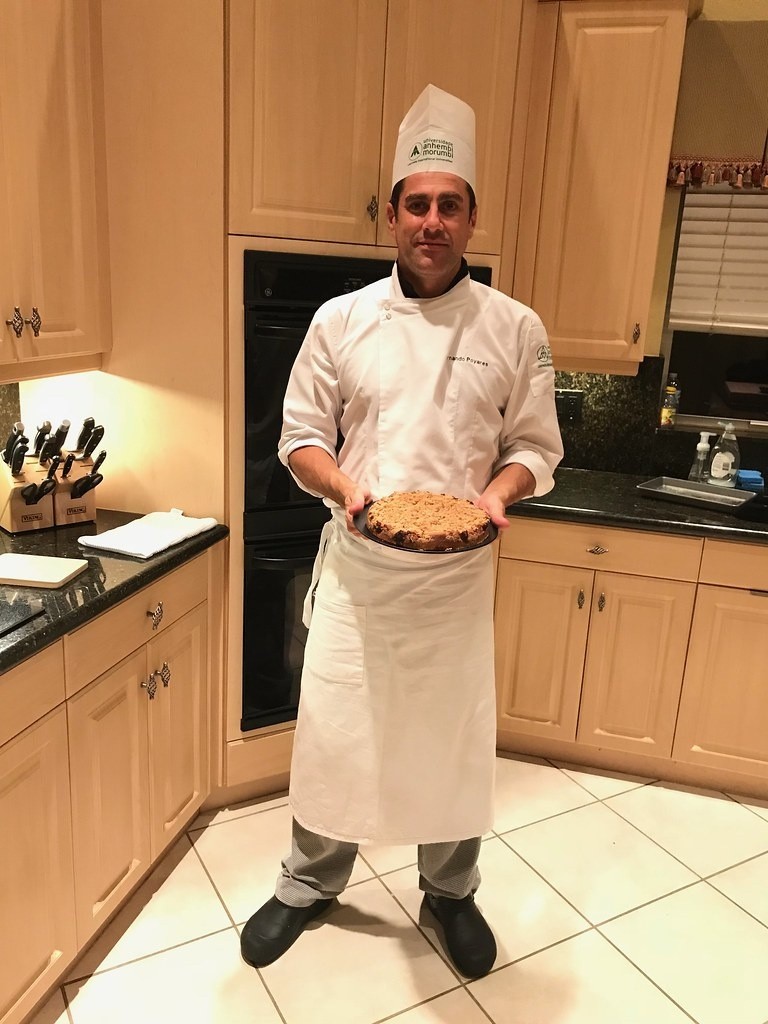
left=239, top=80, right=564, bottom=978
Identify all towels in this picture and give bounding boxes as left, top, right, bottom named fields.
left=78, top=507, right=219, bottom=560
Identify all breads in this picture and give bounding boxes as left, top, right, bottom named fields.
left=366, top=487, right=493, bottom=551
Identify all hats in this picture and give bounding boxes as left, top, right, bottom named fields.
left=390, top=84, right=477, bottom=196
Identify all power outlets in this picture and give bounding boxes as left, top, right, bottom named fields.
left=555, top=389, right=583, bottom=424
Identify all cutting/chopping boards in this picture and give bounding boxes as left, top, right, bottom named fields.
left=0, top=553, right=88, bottom=588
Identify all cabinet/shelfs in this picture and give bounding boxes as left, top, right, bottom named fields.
left=493, top=513, right=768, bottom=803
left=223, top=0, right=706, bottom=381
left=1, top=0, right=114, bottom=389
left=0, top=541, right=226, bottom=1024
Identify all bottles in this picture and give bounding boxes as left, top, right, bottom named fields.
left=707, top=420, right=740, bottom=487
left=660, top=371, right=680, bottom=430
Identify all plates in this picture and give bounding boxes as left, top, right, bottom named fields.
left=351, top=499, right=500, bottom=554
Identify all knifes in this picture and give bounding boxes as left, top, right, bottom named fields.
left=34, top=420, right=59, bottom=479
left=57, top=420, right=75, bottom=478
left=2, top=416, right=30, bottom=478
left=77, top=417, right=107, bottom=475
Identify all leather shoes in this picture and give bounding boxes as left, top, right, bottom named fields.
left=240, top=895, right=334, bottom=969
left=425, top=891, right=496, bottom=979
